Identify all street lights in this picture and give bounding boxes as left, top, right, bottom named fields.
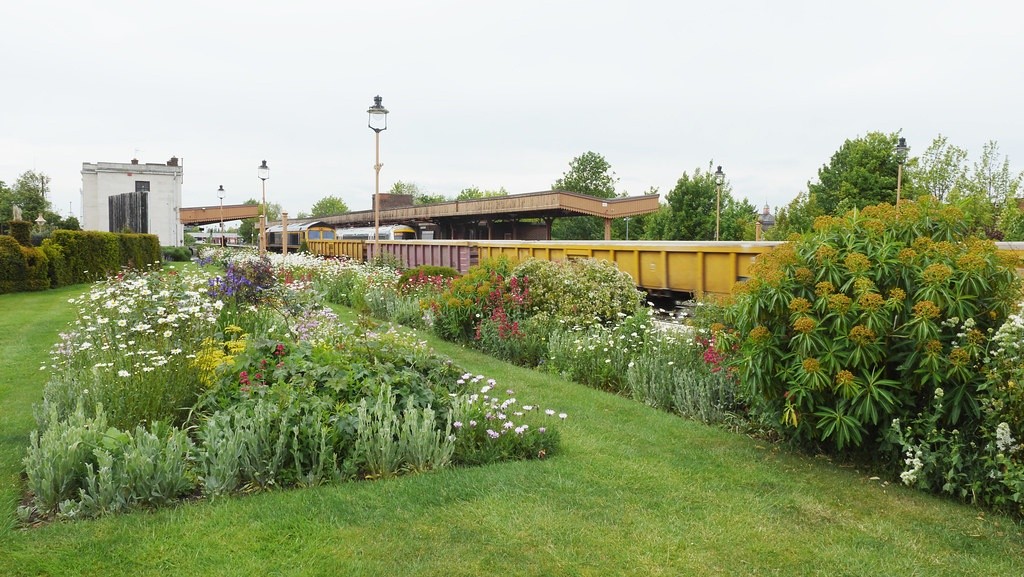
left=259, top=159, right=270, bottom=249
left=894, top=137, right=908, bottom=226
left=713, top=166, right=725, bottom=242
left=215, top=185, right=226, bottom=250
left=624, top=217, right=632, bottom=240
left=35, top=214, right=46, bottom=234
left=368, top=95, right=388, bottom=261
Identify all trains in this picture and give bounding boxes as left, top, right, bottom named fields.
left=335, top=224, right=418, bottom=241
left=258, top=221, right=799, bottom=309
